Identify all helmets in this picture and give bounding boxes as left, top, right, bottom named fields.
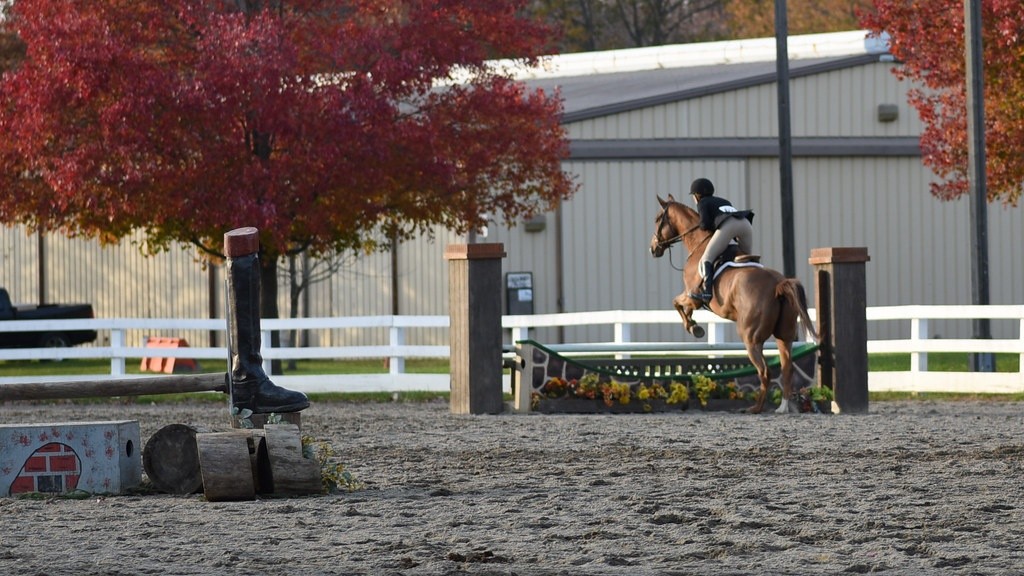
left=689, top=178, right=714, bottom=195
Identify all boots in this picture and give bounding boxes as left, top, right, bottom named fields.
left=225, top=254, right=308, bottom=413
left=690, top=261, right=713, bottom=301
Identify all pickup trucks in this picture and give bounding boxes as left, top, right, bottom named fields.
left=0, top=287, right=99, bottom=346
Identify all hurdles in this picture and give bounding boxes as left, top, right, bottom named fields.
left=443, top=240, right=872, bottom=414
left=0, top=223, right=311, bottom=497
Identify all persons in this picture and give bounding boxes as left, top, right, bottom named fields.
left=224, top=228, right=310, bottom=414
left=687, top=178, right=755, bottom=302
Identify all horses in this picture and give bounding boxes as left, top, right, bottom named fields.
left=649, top=192, right=822, bottom=414
